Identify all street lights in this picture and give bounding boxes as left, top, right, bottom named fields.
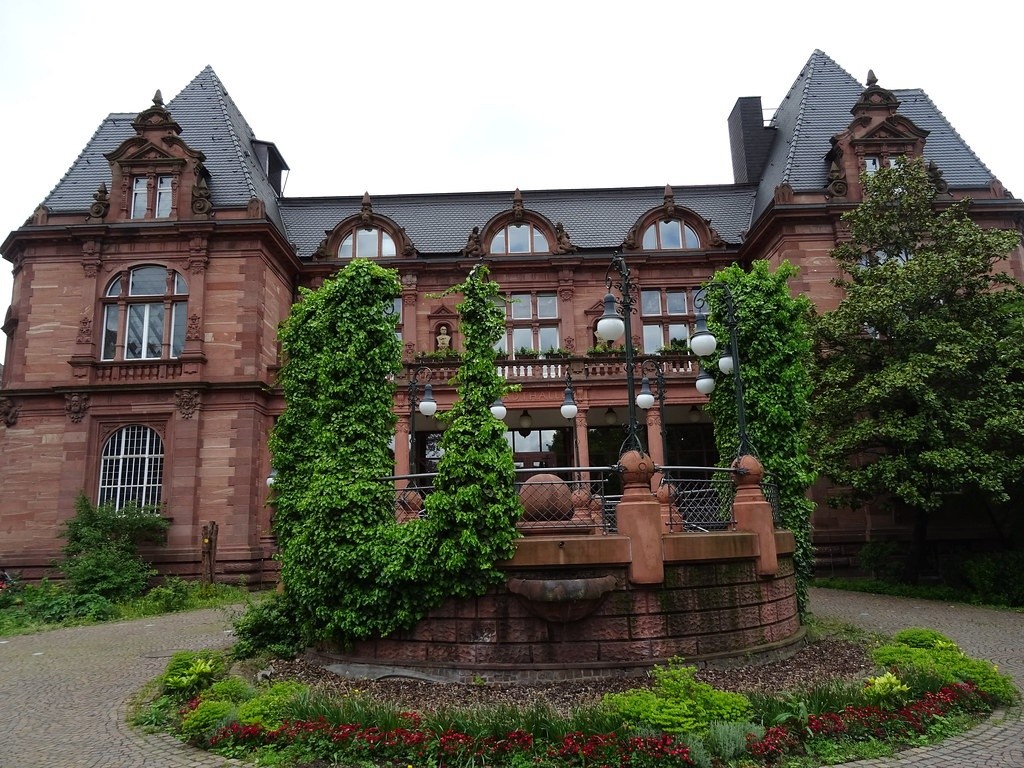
left=635, top=354, right=677, bottom=505
left=559, top=364, right=592, bottom=508
left=402, top=358, right=438, bottom=512
left=690, top=282, right=764, bottom=487
left=597, top=250, right=654, bottom=489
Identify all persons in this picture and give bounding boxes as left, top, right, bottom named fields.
left=551, top=222, right=577, bottom=256
left=70, top=396, right=81, bottom=413
left=0, top=397, right=12, bottom=424
left=178, top=392, right=195, bottom=409
left=437, top=326, right=451, bottom=348
left=462, top=227, right=481, bottom=258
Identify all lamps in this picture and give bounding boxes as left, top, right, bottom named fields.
left=688, top=406, right=702, bottom=423
left=435, top=412, right=447, bottom=431
left=520, top=410, right=533, bottom=428
left=603, top=408, right=619, bottom=424
